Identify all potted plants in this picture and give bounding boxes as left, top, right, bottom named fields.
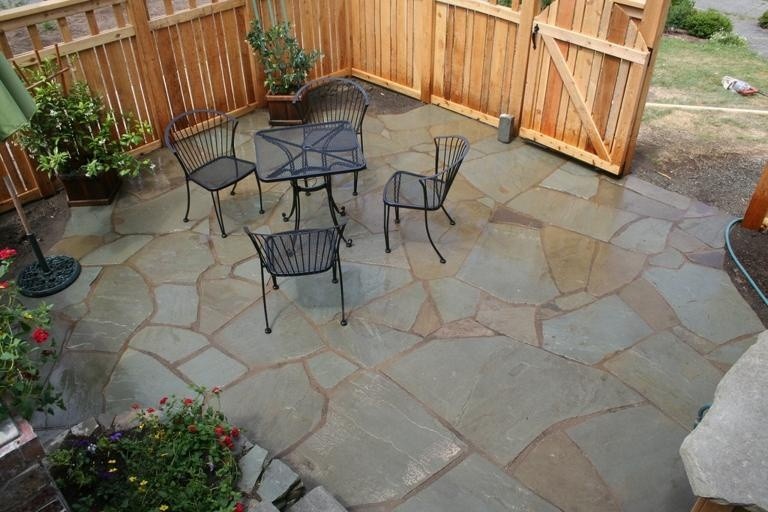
left=244, top=16, right=322, bottom=127
left=8, top=54, right=159, bottom=208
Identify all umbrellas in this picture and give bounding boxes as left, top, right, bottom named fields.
left=1, top=51, right=50, bottom=272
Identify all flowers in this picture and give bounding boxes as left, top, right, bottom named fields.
left=0, top=246, right=65, bottom=418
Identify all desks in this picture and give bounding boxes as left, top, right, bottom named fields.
left=252, top=119, right=368, bottom=257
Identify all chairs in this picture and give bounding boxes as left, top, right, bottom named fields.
left=244, top=222, right=349, bottom=335
left=381, top=135, right=471, bottom=265
left=291, top=75, right=370, bottom=197
left=164, top=108, right=265, bottom=240
